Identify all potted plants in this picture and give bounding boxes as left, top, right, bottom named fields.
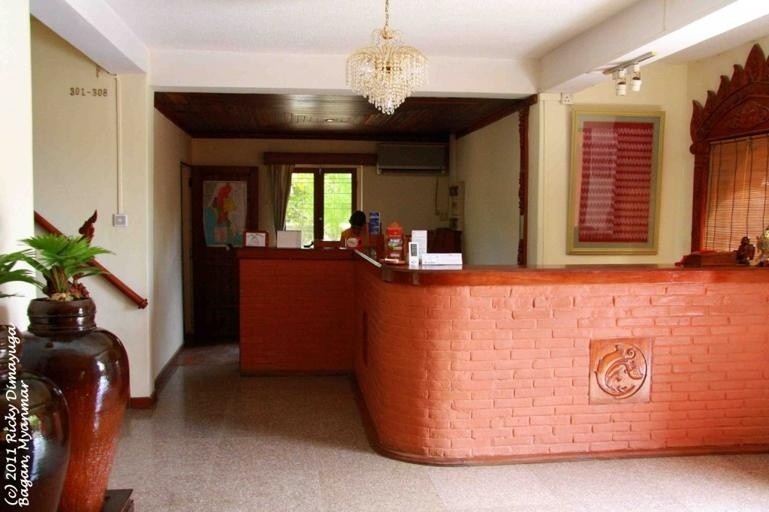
left=0, top=249, right=76, bottom=512
left=19, top=226, right=133, bottom=512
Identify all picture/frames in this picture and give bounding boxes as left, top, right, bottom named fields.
left=562, top=101, right=668, bottom=258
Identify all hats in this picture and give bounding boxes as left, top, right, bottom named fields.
left=348, top=210, right=367, bottom=226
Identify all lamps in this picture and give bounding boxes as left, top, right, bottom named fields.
left=611, top=61, right=642, bottom=96
left=342, top=1, right=432, bottom=121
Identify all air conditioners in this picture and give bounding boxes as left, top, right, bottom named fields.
left=374, top=141, right=449, bottom=176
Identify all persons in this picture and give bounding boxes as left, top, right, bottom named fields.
left=341, top=212, right=370, bottom=248
